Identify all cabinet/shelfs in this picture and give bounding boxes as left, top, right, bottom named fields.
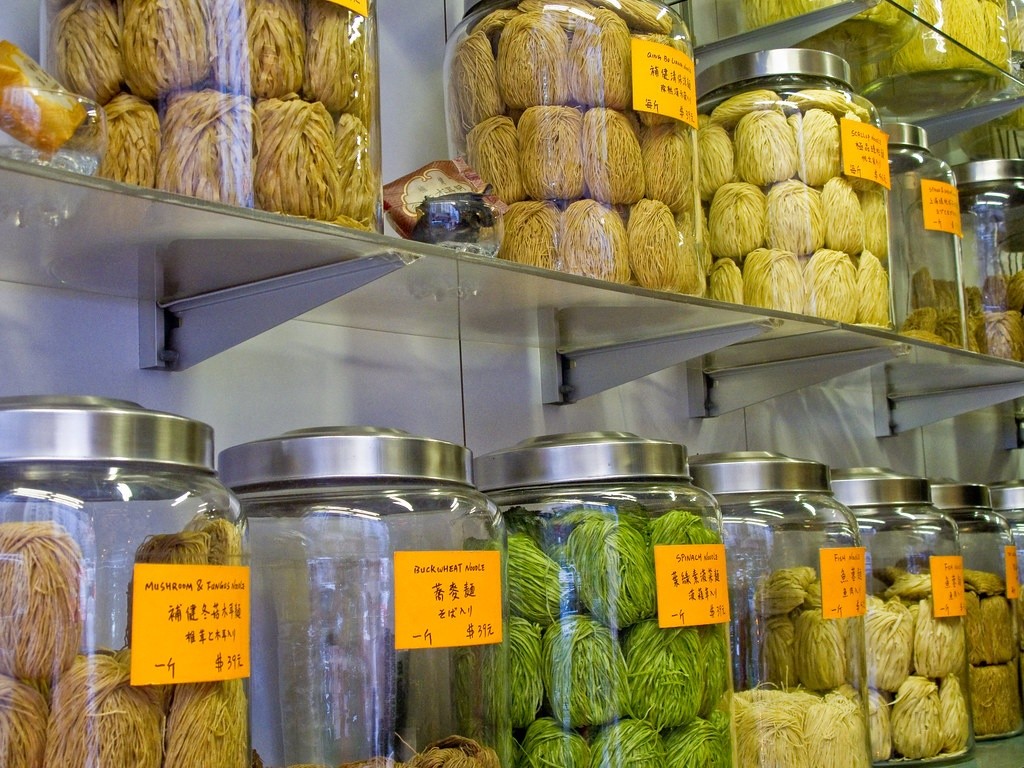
left=0, top=0, right=1024, bottom=768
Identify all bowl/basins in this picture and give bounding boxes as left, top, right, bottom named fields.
left=0, top=86, right=106, bottom=177
left=385, top=198, right=505, bottom=258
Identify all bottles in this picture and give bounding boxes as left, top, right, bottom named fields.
left=0, top=393, right=1024, bottom=768
left=442, top=0, right=708, bottom=298
left=696, top=0, right=1024, bottom=363
left=38, top=0, right=383, bottom=235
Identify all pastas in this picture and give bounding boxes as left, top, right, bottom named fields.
left=42, top=0, right=1024, bottom=365
left=0, top=504, right=1024, bottom=768
